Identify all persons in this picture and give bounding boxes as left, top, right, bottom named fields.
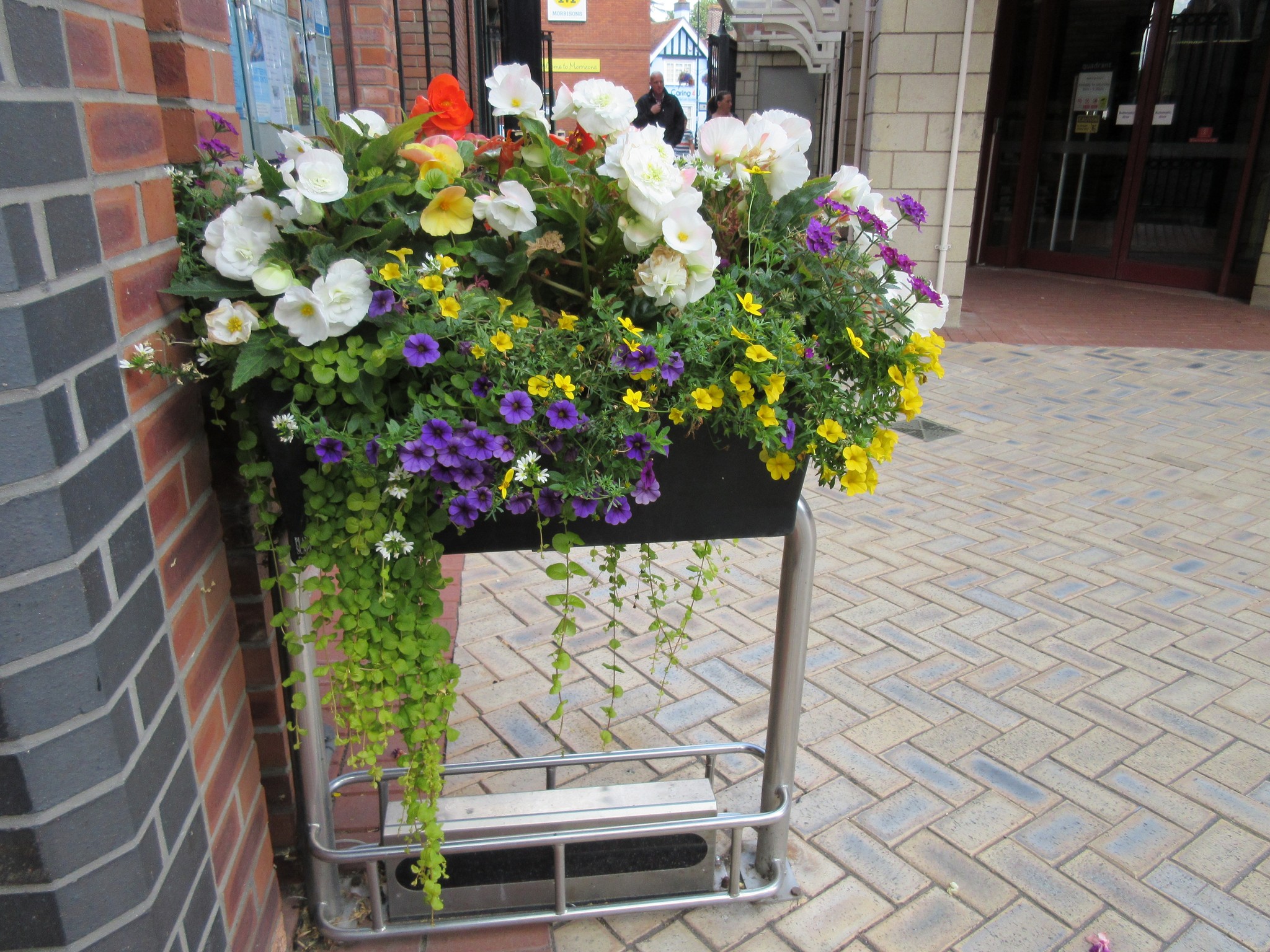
left=704, top=90, right=739, bottom=123
left=632, top=70, right=685, bottom=148
left=672, top=114, right=696, bottom=158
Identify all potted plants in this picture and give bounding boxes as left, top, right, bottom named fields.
left=106, top=64, right=956, bottom=921
